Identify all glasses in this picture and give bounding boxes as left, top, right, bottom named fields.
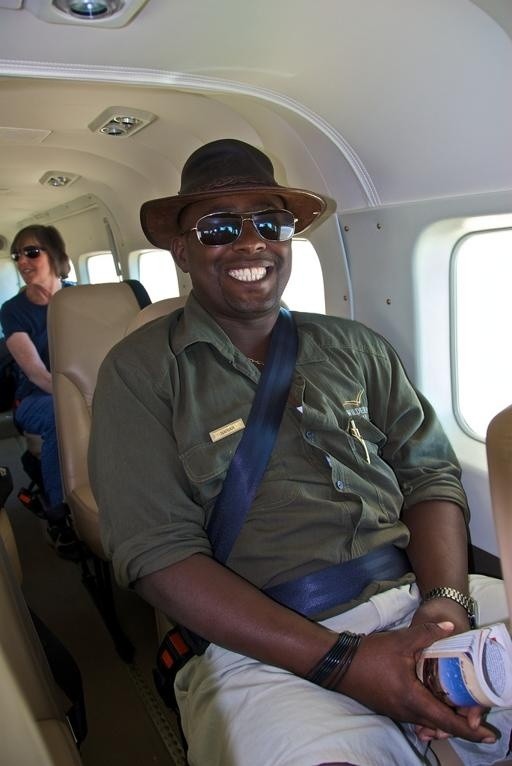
left=11, top=247, right=46, bottom=261
left=181, top=207, right=299, bottom=248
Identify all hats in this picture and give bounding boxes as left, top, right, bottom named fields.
left=140, top=139, right=336, bottom=250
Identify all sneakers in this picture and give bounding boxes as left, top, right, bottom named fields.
left=46, top=504, right=79, bottom=552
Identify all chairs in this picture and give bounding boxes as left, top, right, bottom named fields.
left=45, top=277, right=157, bottom=668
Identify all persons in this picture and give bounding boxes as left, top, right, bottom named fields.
left=90, top=137, right=510, bottom=766
left=1, top=223, right=83, bottom=554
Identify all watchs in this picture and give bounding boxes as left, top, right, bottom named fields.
left=421, top=587, right=480, bottom=626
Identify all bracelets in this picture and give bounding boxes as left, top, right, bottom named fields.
left=304, top=632, right=362, bottom=693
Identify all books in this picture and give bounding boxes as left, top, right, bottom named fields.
left=414, top=621, right=510, bottom=709
